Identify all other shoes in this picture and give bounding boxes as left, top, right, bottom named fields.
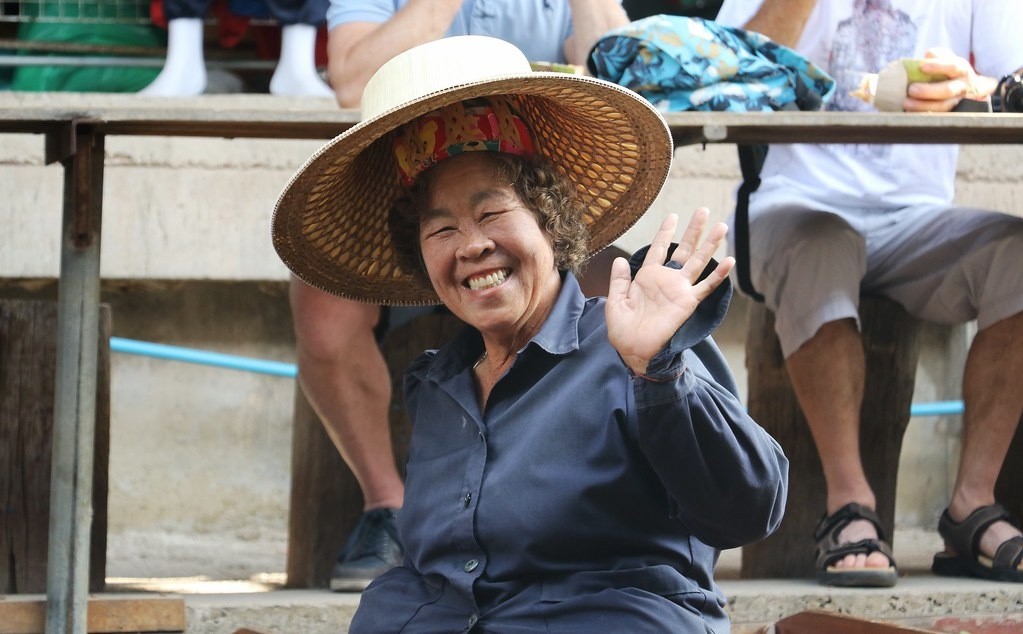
left=327, top=500, right=406, bottom=593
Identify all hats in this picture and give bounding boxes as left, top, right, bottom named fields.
left=267, top=34, right=676, bottom=308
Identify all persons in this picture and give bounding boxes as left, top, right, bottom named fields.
left=714, top=1, right=1023, bottom=588
left=136, top=0, right=336, bottom=97
left=289, top=1, right=631, bottom=592
left=270, top=34, right=789, bottom=634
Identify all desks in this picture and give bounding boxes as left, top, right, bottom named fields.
left=0, top=85, right=1023, bottom=630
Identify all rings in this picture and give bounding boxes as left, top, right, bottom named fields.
left=664, top=261, right=682, bottom=269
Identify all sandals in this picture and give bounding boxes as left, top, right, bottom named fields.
left=931, top=503, right=1023, bottom=582
left=811, top=502, right=899, bottom=588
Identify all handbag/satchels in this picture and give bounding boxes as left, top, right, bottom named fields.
left=582, top=13, right=839, bottom=115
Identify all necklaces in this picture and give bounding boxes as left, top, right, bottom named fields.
left=473, top=352, right=486, bottom=372
